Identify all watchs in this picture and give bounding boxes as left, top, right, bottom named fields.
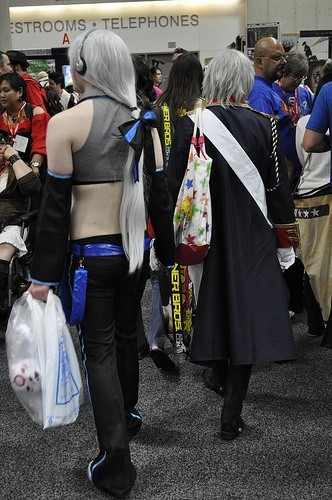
left=30, top=161, right=40, bottom=168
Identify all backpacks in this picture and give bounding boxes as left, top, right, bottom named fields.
left=24, top=78, right=64, bottom=116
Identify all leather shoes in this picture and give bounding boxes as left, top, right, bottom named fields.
left=203, top=368, right=226, bottom=398
left=221, top=420, right=244, bottom=438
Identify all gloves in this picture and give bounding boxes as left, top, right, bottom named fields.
left=277, top=246, right=296, bottom=272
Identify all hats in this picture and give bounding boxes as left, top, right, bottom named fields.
left=38, top=71, right=49, bottom=81
left=7, top=50, right=30, bottom=67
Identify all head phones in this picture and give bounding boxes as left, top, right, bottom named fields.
left=75, top=27, right=96, bottom=74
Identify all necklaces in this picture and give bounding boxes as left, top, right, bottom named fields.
left=7, top=110, right=18, bottom=123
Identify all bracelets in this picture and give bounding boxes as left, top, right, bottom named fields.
left=9, top=154, right=21, bottom=165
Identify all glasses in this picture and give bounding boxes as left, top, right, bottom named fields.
left=257, top=54, right=289, bottom=62
left=288, top=75, right=308, bottom=82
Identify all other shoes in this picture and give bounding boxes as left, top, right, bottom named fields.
left=150, top=349, right=176, bottom=369
left=307, top=326, right=323, bottom=336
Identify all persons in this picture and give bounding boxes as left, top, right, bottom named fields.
left=29, top=28, right=175, bottom=500
left=144, top=50, right=204, bottom=373
left=131, top=53, right=157, bottom=359
left=246, top=37, right=332, bottom=351
left=0, top=48, right=77, bottom=347
left=169, top=49, right=297, bottom=442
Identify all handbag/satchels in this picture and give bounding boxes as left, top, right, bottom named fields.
left=171, top=107, right=213, bottom=265
left=4, top=288, right=82, bottom=430
